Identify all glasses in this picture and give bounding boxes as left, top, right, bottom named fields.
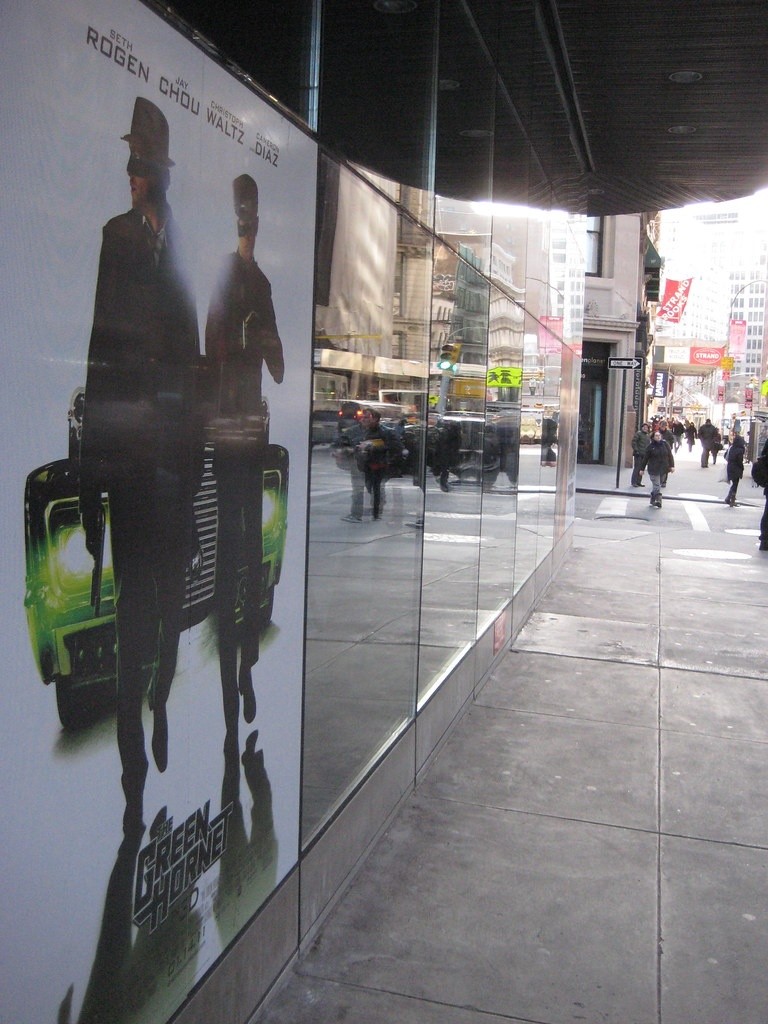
left=127, top=153, right=161, bottom=178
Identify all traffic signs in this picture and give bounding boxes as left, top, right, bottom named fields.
left=608, top=357, right=643, bottom=370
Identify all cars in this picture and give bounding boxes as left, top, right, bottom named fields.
left=311, top=409, right=342, bottom=448
left=24, top=355, right=288, bottom=729
left=723, top=418, right=754, bottom=444
left=521, top=409, right=543, bottom=444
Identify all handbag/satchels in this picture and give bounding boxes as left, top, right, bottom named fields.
left=715, top=442, right=723, bottom=450
left=717, top=462, right=731, bottom=485
left=751, top=455, right=768, bottom=487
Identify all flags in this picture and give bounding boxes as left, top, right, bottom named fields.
left=657, top=278, right=692, bottom=323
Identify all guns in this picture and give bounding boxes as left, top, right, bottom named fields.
left=81, top=501, right=108, bottom=620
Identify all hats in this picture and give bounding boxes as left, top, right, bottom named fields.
left=120, top=97, right=176, bottom=166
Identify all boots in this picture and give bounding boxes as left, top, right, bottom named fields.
left=650, top=492, right=657, bottom=506
left=656, top=493, right=662, bottom=507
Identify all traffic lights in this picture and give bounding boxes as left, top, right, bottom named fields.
left=750, top=378, right=754, bottom=384
left=754, top=378, right=759, bottom=387
left=436, top=343, right=461, bottom=370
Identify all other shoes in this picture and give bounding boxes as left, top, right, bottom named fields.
left=238, top=665, right=256, bottom=724
left=637, top=483, right=645, bottom=487
left=661, top=482, right=666, bottom=487
left=631, top=482, right=638, bottom=487
left=343, top=514, right=363, bottom=523
left=223, top=696, right=238, bottom=736
left=725, top=495, right=731, bottom=503
left=146, top=678, right=168, bottom=772
left=730, top=498, right=737, bottom=507
left=759, top=541, right=768, bottom=550
left=115, top=709, right=149, bottom=774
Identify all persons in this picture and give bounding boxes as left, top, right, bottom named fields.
left=205, top=172, right=285, bottom=739
left=629, top=415, right=722, bottom=508
left=78, top=98, right=210, bottom=846
left=335, top=401, right=557, bottom=522
left=724, top=436, right=747, bottom=507
left=752, top=439, right=768, bottom=549
